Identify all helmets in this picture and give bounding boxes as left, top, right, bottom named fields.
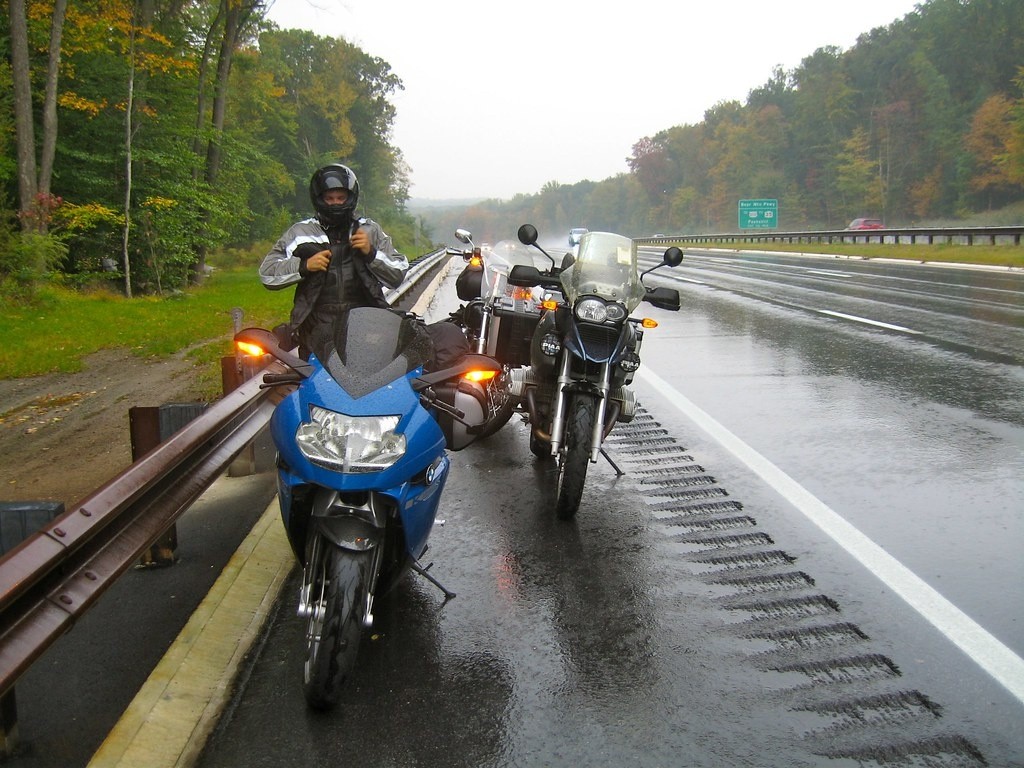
left=309, top=163, right=360, bottom=222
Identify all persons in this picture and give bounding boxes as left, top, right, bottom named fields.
left=258, top=164, right=410, bottom=366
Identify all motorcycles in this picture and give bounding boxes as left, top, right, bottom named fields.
left=234, top=305, right=503, bottom=717
left=445, top=223, right=685, bottom=524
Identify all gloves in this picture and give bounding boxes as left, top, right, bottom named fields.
left=307, top=250, right=332, bottom=272
left=350, top=228, right=370, bottom=256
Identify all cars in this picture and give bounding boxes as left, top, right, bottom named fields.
left=843, top=218, right=885, bottom=230
left=653, top=234, right=665, bottom=237
left=568, top=228, right=589, bottom=248
